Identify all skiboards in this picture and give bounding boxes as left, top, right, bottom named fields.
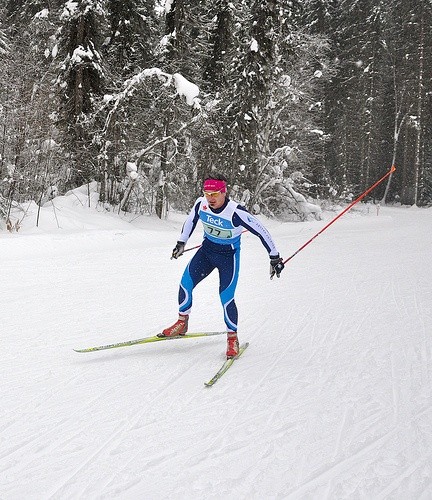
left=70, top=329, right=249, bottom=388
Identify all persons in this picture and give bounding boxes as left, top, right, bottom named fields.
left=161, top=172, right=286, bottom=359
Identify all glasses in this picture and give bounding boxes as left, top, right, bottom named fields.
left=202, top=188, right=224, bottom=197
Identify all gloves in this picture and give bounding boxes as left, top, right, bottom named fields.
left=172, top=241, right=185, bottom=259
left=268, top=254, right=284, bottom=280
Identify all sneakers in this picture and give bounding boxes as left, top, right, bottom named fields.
left=160, top=315, right=189, bottom=336
left=225, top=331, right=239, bottom=358
